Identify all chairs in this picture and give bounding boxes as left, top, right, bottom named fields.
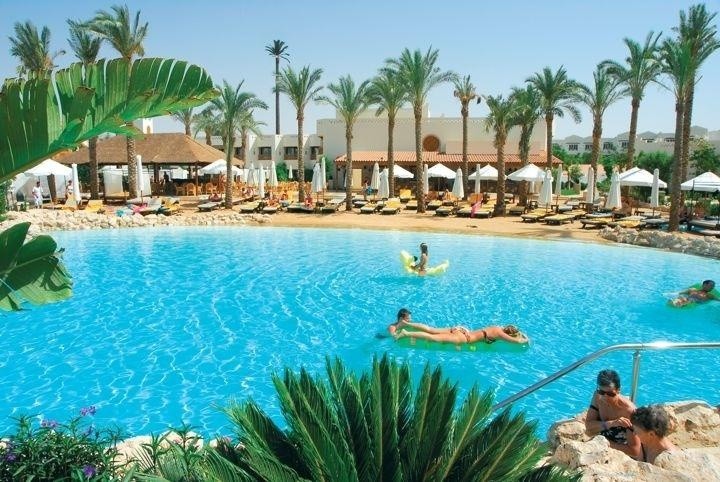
left=58, top=180, right=342, bottom=213
left=538, top=198, right=717, bottom=236
left=349, top=187, right=532, bottom=218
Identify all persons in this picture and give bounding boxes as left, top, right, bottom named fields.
left=32, top=181, right=44, bottom=209
left=391, top=319, right=531, bottom=346
left=410, top=241, right=428, bottom=276
left=163, top=170, right=170, bottom=184
left=387, top=307, right=412, bottom=334
left=583, top=368, right=644, bottom=463
left=678, top=198, right=689, bottom=224
left=206, top=189, right=313, bottom=209
left=630, top=405, right=677, bottom=464
left=668, top=279, right=720, bottom=309
left=361, top=179, right=369, bottom=201
left=64, top=180, right=73, bottom=199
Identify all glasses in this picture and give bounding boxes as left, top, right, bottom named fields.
left=596, top=387, right=619, bottom=398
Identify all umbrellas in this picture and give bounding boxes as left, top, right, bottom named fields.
left=246, top=159, right=278, bottom=198
left=507, top=163, right=553, bottom=205
left=527, top=181, right=535, bottom=203
left=681, top=171, right=720, bottom=199
left=537, top=169, right=553, bottom=213
left=24, top=157, right=72, bottom=177
left=136, top=155, right=145, bottom=206
left=320, top=157, right=326, bottom=203
left=310, top=162, right=323, bottom=203
left=288, top=163, right=293, bottom=179
left=200, top=157, right=243, bottom=199
left=371, top=161, right=380, bottom=198
left=554, top=163, right=562, bottom=205
left=604, top=166, right=622, bottom=222
left=650, top=168, right=660, bottom=217
left=377, top=167, right=389, bottom=205
left=585, top=166, right=594, bottom=214
left=619, top=166, right=640, bottom=178
left=70, top=162, right=82, bottom=202
left=388, top=164, right=414, bottom=179
left=621, top=169, right=666, bottom=210
left=423, top=162, right=456, bottom=196
left=451, top=166, right=465, bottom=211
